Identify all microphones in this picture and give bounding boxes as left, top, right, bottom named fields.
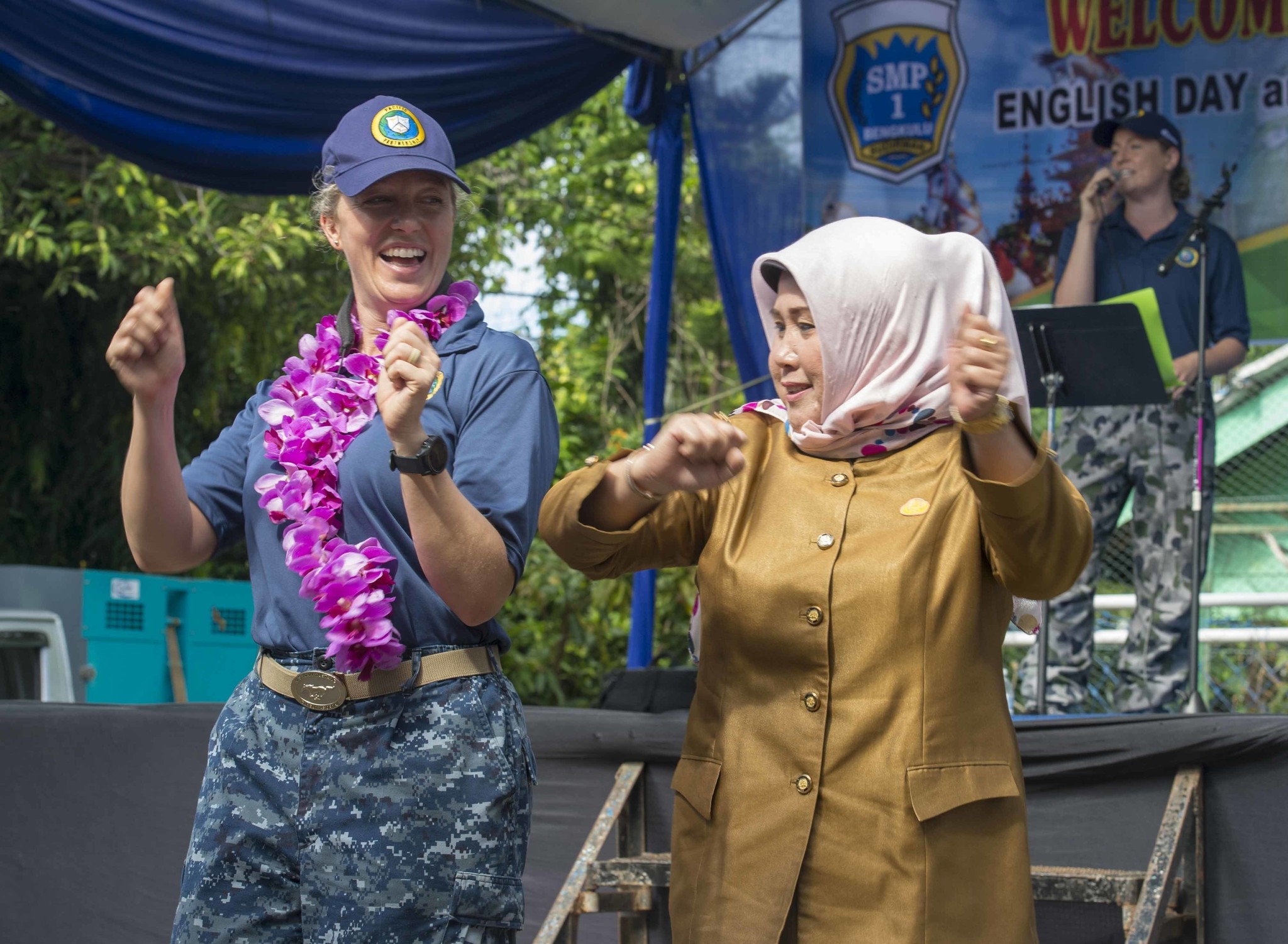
left=1095, top=169, right=1120, bottom=194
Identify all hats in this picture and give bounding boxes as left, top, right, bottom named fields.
left=322, top=94, right=473, bottom=197
left=1091, top=111, right=1183, bottom=153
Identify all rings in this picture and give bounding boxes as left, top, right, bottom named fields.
left=980, top=335, right=1000, bottom=350
left=408, top=347, right=422, bottom=365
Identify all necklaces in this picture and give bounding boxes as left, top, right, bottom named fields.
left=254, top=278, right=481, bottom=673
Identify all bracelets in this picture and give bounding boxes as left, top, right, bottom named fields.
left=948, top=394, right=1015, bottom=436
left=623, top=443, right=671, bottom=503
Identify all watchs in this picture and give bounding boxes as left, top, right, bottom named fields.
left=388, top=435, right=450, bottom=477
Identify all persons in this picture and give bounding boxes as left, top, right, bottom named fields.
left=106, top=95, right=554, bottom=944
left=535, top=215, right=1099, bottom=944
left=1004, top=112, right=1254, bottom=714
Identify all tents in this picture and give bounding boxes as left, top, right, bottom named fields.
left=1, top=0, right=1287, bottom=704
